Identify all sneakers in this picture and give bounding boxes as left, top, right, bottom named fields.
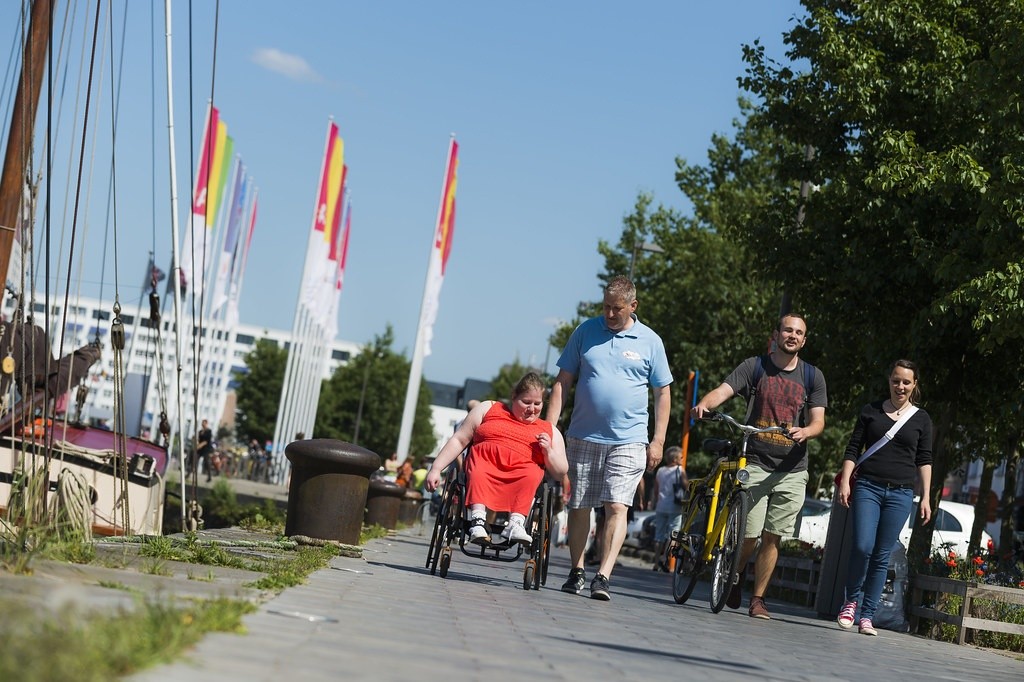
left=749, top=600, right=770, bottom=620
left=837, top=600, right=858, bottom=629
left=469, top=525, right=487, bottom=540
left=501, top=519, right=532, bottom=544
left=725, top=573, right=743, bottom=609
left=590, top=576, right=610, bottom=600
left=858, top=617, right=877, bottom=636
left=561, top=570, right=587, bottom=593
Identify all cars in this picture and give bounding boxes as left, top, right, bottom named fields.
left=552, top=495, right=833, bottom=552
left=780, top=493, right=994, bottom=566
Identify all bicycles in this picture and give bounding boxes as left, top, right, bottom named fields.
left=663, top=409, right=803, bottom=614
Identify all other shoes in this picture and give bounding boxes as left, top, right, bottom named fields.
left=652, top=567, right=658, bottom=572
left=586, top=557, right=601, bottom=566
left=613, top=561, right=621, bottom=568
left=657, top=558, right=670, bottom=572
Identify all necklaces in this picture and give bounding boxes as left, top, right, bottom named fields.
left=890, top=400, right=909, bottom=415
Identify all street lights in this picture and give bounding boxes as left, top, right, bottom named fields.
left=354, top=341, right=384, bottom=445
left=576, top=300, right=595, bottom=326
left=629, top=238, right=665, bottom=280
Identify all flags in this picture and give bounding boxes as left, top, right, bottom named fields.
left=420, top=141, right=460, bottom=356
left=297, top=120, right=352, bottom=338
left=185, top=107, right=257, bottom=331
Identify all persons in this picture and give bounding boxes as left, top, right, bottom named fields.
left=250, top=439, right=272, bottom=461
left=535, top=427, right=646, bottom=567
left=690, top=313, right=828, bottom=619
left=384, top=453, right=428, bottom=492
left=837, top=361, right=933, bottom=635
left=185, top=420, right=213, bottom=482
left=546, top=275, right=674, bottom=600
left=653, top=446, right=688, bottom=574
left=426, top=372, right=569, bottom=545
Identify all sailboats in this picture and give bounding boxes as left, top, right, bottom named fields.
left=1, top=1, right=224, bottom=539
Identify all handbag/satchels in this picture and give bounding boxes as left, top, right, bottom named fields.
left=673, top=467, right=685, bottom=506
left=834, top=460, right=860, bottom=501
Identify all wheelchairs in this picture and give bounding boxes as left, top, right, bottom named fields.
left=424, top=418, right=563, bottom=592
